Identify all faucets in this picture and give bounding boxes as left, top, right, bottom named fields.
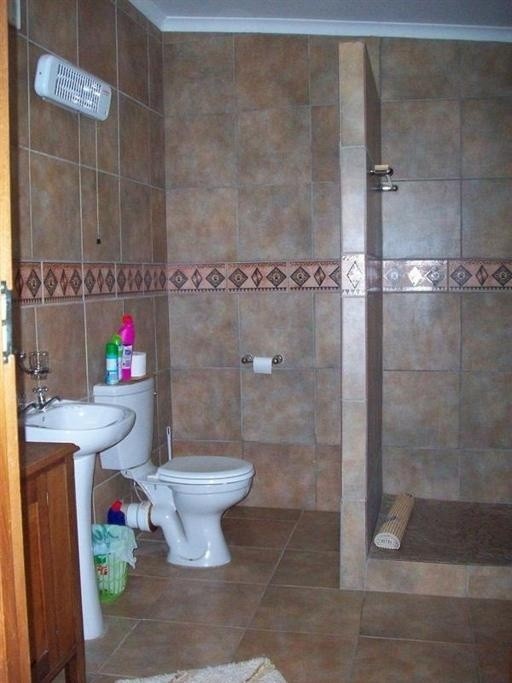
left=20, top=402, right=38, bottom=418
left=39, top=394, right=62, bottom=412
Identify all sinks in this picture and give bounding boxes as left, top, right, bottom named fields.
left=22, top=399, right=136, bottom=454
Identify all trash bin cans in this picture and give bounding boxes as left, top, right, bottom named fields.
left=92, top=522, right=134, bottom=605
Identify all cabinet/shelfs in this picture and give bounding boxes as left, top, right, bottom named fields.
left=18, top=441, right=88, bottom=683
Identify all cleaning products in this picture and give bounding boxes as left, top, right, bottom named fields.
left=104, top=313, right=135, bottom=386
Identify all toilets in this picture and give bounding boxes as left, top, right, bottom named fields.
left=92, top=377, right=256, bottom=569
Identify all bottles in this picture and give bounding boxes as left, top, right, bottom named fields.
left=105, top=313, right=135, bottom=385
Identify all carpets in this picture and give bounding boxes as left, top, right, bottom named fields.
left=114, top=655, right=288, bottom=683
